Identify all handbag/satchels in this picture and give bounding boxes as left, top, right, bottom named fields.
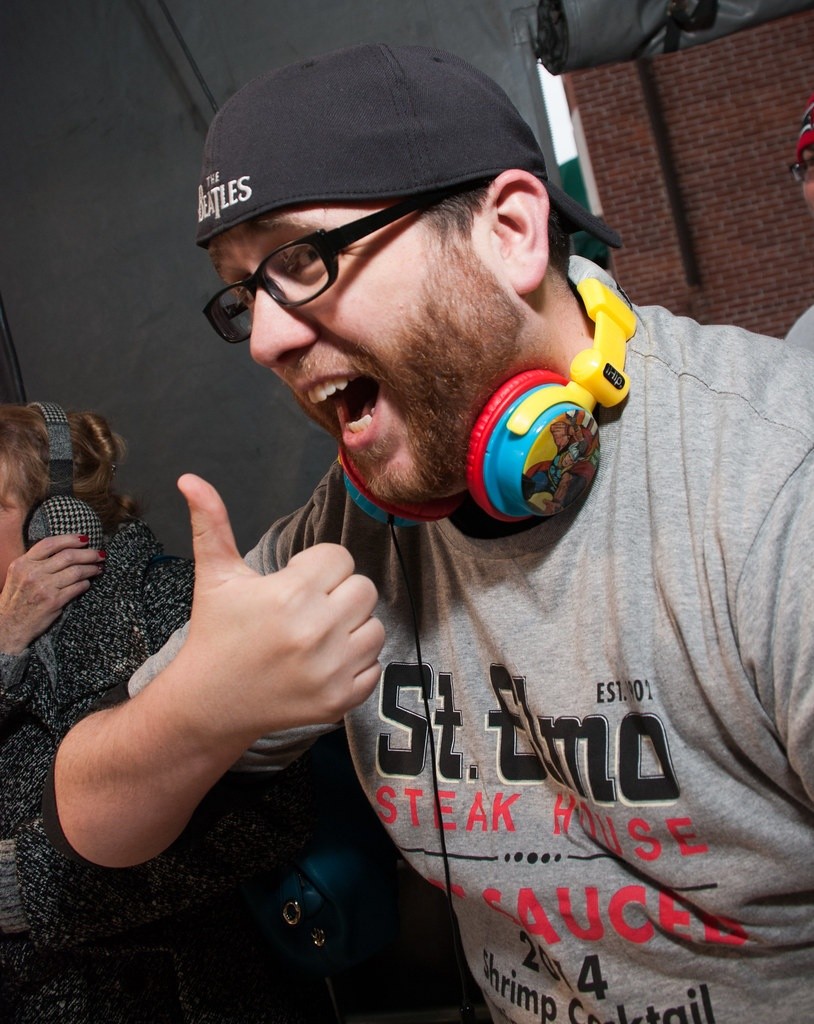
left=240, top=821, right=403, bottom=985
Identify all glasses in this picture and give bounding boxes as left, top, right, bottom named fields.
left=791, top=158, right=814, bottom=183
left=204, top=175, right=499, bottom=345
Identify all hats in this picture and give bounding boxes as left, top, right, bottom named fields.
left=197, top=41, right=622, bottom=248
left=795, top=94, right=814, bottom=162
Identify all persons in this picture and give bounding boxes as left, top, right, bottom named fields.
left=784, top=90, right=814, bottom=350
left=56, top=41, right=813, bottom=1024
left=0, top=400, right=314, bottom=1024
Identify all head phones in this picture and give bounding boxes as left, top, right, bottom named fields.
left=21, top=401, right=103, bottom=556
left=332, top=255, right=638, bottom=529
